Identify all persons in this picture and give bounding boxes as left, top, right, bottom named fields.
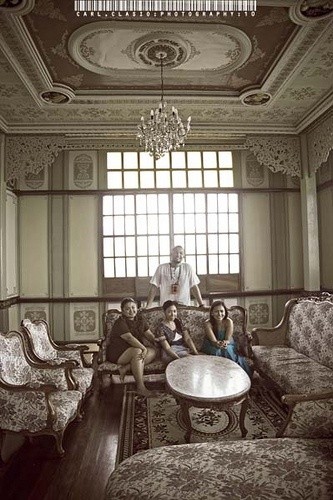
left=202, top=301, right=245, bottom=367
left=106, top=297, right=160, bottom=398
left=138, top=245, right=205, bottom=309
left=155, top=300, right=206, bottom=363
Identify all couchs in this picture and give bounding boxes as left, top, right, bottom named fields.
left=251, top=290, right=333, bottom=438
left=20, top=318, right=96, bottom=417
left=1, top=330, right=84, bottom=456
left=95, top=305, right=254, bottom=399
left=104, top=438, right=333, bottom=499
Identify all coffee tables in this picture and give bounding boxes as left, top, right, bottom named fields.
left=164, top=355, right=252, bottom=444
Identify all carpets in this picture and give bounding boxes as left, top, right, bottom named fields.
left=114, top=382, right=290, bottom=469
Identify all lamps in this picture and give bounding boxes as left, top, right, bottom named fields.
left=134, top=55, right=193, bottom=160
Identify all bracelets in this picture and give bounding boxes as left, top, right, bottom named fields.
left=199, top=304, right=205, bottom=307
